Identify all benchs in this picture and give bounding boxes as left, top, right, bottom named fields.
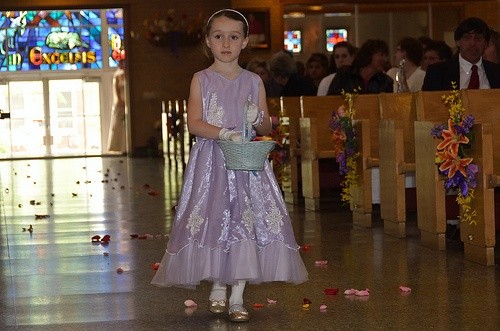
left=267, top=87, right=500, bottom=268
left=163, top=99, right=195, bottom=167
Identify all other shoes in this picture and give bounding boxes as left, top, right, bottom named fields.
left=207, top=288, right=228, bottom=313
left=228, top=304, right=249, bottom=322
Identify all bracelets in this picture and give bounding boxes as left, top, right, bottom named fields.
left=219, top=128, right=237, bottom=141
left=252, top=111, right=265, bottom=127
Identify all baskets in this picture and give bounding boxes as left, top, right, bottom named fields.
left=214, top=109, right=276, bottom=171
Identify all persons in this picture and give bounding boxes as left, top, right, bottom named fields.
left=247, top=17, right=500, bottom=170
left=152, top=10, right=309, bottom=324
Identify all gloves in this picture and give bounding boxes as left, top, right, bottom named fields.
left=218, top=126, right=248, bottom=142
left=245, top=104, right=261, bottom=125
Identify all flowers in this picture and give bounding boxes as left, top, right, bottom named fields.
left=329, top=87, right=361, bottom=211
left=428, top=79, right=478, bottom=226
left=269, top=97, right=290, bottom=190
left=165, top=109, right=185, bottom=144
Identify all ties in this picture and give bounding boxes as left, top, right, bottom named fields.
left=468, top=64, right=480, bottom=89
